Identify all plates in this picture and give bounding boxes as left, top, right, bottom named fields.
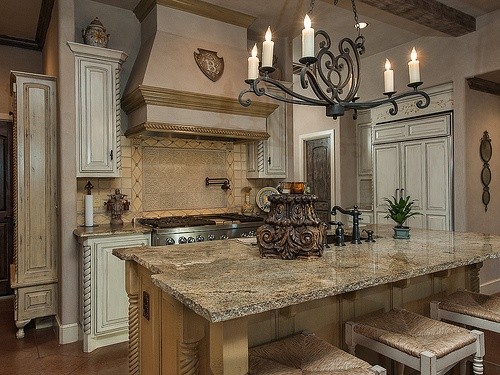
left=256, top=186, right=281, bottom=212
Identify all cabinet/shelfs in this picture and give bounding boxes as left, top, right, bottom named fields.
left=246, top=80, right=293, bottom=179
left=91, top=238, right=149, bottom=338
left=67, top=40, right=129, bottom=178
left=8, top=69, right=57, bottom=339
left=371, top=82, right=453, bottom=231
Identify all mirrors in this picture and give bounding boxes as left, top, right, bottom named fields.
left=480, top=130, right=493, bottom=212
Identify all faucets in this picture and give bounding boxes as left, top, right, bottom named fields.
left=330, top=205, right=363, bottom=245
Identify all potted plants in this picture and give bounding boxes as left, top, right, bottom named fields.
left=382, top=196, right=424, bottom=239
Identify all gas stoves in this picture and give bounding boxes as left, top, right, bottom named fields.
left=137, top=211, right=264, bottom=246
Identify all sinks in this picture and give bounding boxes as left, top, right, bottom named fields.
left=320, top=234, right=368, bottom=247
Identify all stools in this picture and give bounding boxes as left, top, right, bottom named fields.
left=248, top=330, right=387, bottom=375
left=429, top=288, right=500, bottom=374
left=345, top=306, right=485, bottom=375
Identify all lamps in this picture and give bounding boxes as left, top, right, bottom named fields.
left=238, top=1, right=430, bottom=120
